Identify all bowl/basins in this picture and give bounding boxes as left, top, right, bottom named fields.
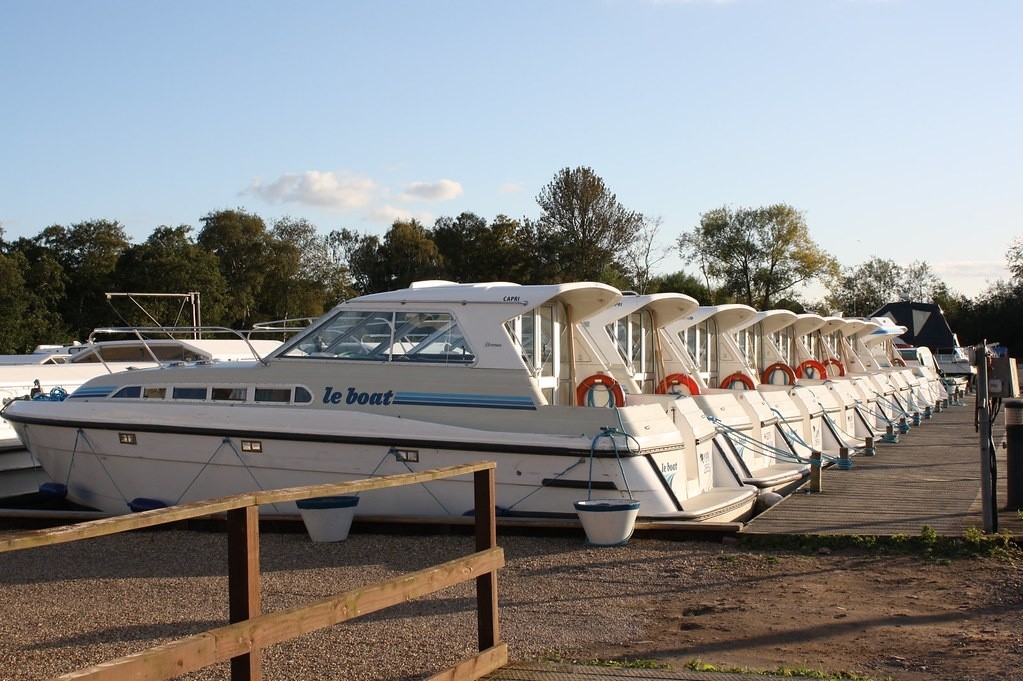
left=296, top=497, right=358, bottom=543
left=573, top=498, right=641, bottom=546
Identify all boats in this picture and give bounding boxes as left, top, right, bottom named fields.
left=1, top=290, right=1004, bottom=503
left=0, top=279, right=762, bottom=524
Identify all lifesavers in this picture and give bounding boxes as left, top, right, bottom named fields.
left=893, top=343, right=914, bottom=348
left=821, top=358, right=844, bottom=376
left=577, top=374, right=625, bottom=408
left=795, top=360, right=827, bottom=379
left=656, top=373, right=701, bottom=395
left=720, top=373, right=756, bottom=390
left=761, top=363, right=797, bottom=385
